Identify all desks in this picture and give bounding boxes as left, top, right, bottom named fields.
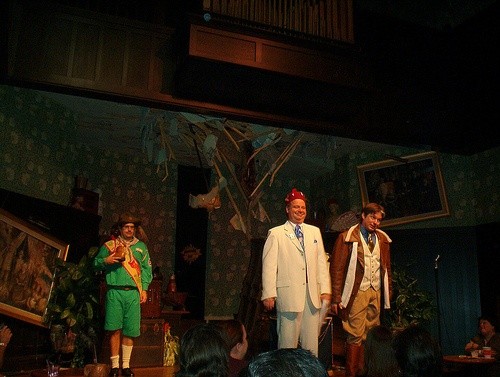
left=443, top=354, right=499, bottom=377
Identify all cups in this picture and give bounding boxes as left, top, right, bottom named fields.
left=471, top=349, right=478, bottom=357
left=47, top=360, right=59, bottom=377
left=483, top=347, right=491, bottom=358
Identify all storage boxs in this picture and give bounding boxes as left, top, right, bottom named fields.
left=99, top=275, right=167, bottom=367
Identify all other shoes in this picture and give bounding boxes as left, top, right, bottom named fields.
left=121, top=368, right=134, bottom=377
left=109, top=368, right=120, bottom=377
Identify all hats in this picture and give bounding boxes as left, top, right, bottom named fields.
left=112, top=212, right=142, bottom=229
left=286, top=189, right=306, bottom=205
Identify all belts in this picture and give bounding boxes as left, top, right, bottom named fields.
left=108, top=286, right=136, bottom=291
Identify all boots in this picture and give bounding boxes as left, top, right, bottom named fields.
left=345, top=343, right=358, bottom=377
left=358, top=342, right=365, bottom=373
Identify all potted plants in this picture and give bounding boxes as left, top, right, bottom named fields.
left=386, top=261, right=440, bottom=336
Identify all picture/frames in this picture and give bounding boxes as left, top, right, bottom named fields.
left=358, top=150, right=449, bottom=228
left=0, top=209, right=71, bottom=330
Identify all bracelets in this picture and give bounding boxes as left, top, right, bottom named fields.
left=0, top=343, right=7, bottom=347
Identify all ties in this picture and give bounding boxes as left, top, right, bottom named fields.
left=367, top=234, right=374, bottom=253
left=295, top=226, right=304, bottom=249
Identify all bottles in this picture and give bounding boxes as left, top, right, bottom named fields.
left=168, top=274, right=177, bottom=300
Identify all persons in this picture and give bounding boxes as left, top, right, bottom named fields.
left=174, top=318, right=444, bottom=377
left=262, top=188, right=331, bottom=358
left=93, top=212, right=153, bottom=377
left=0, top=325, right=13, bottom=368
left=331, top=202, right=393, bottom=377
left=464, top=316, right=500, bottom=361
